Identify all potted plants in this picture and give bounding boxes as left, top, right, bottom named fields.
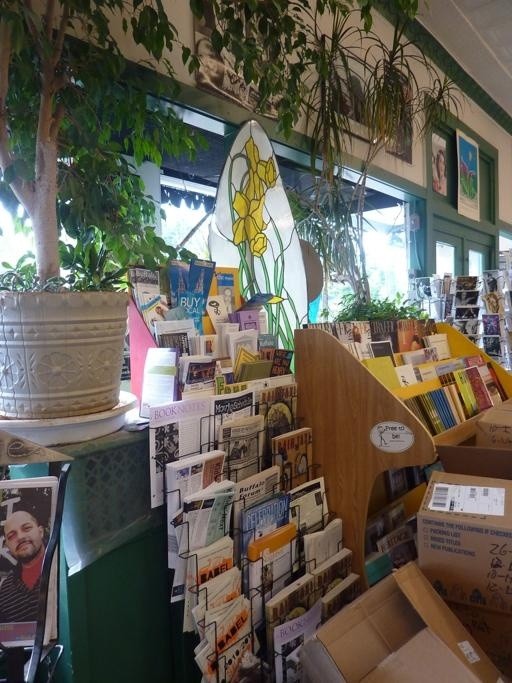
left=320, top=33, right=386, bottom=142
left=0, top=0, right=214, bottom=420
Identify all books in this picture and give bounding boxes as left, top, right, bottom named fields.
left=0, top=474, right=60, bottom=649
left=415, top=270, right=511, bottom=370
left=302, top=319, right=507, bottom=585
left=128, top=255, right=359, bottom=683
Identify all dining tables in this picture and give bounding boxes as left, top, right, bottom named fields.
left=0, top=0, right=214, bottom=420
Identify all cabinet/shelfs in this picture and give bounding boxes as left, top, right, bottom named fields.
left=124, top=298, right=512, bottom=683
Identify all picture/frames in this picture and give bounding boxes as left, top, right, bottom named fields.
left=9, top=378, right=202, bottom=683
left=320, top=33, right=386, bottom=142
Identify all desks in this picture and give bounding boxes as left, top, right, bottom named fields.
left=9, top=378, right=202, bottom=683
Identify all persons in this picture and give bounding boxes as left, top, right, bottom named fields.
left=436, top=149, right=446, bottom=194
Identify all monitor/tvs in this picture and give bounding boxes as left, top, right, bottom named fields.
left=0, top=474, right=60, bottom=649
